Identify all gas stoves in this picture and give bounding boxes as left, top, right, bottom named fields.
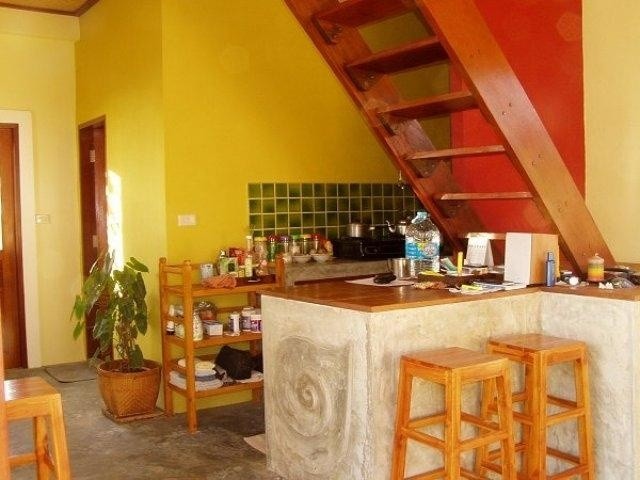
left=334, top=239, right=405, bottom=257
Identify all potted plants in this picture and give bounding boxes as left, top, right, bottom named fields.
left=67, top=246, right=161, bottom=417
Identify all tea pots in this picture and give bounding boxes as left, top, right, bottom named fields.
left=385, top=220, right=410, bottom=236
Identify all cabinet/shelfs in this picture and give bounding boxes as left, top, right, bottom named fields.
left=158, top=256, right=286, bottom=430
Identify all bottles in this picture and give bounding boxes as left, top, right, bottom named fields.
left=267, top=236, right=281, bottom=262
left=290, top=234, right=300, bottom=254
left=166, top=306, right=176, bottom=335
left=312, top=233, right=321, bottom=250
left=300, top=234, right=312, bottom=254
left=281, top=235, right=290, bottom=253
left=244, top=236, right=257, bottom=280
left=406, top=211, right=440, bottom=276
left=254, top=237, right=267, bottom=263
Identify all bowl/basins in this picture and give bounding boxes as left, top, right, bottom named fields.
left=292, top=255, right=311, bottom=263
left=444, top=273, right=475, bottom=288
left=311, top=254, right=330, bottom=263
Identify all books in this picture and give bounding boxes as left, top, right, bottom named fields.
left=472, top=281, right=528, bottom=291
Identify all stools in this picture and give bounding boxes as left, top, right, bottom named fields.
left=473, top=334, right=595, bottom=480
left=3, top=375, right=71, bottom=480
left=390, top=346, right=517, bottom=480
